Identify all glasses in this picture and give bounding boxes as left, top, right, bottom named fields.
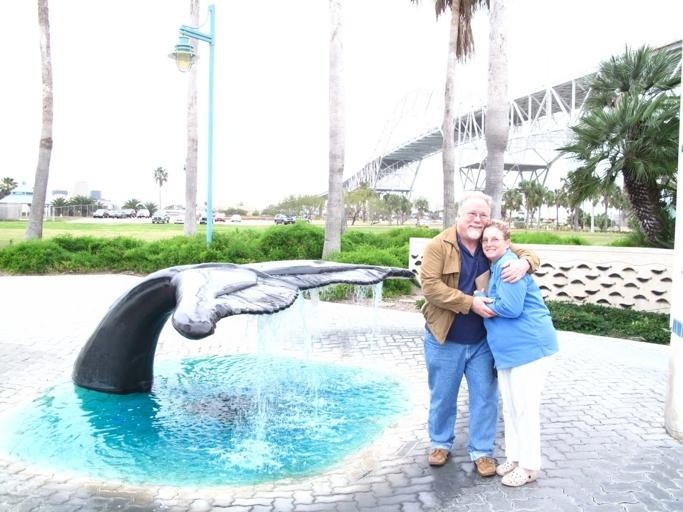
left=480, top=238, right=507, bottom=243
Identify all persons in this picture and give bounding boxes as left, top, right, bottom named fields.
left=472, top=218, right=561, bottom=487
left=418, top=190, right=541, bottom=480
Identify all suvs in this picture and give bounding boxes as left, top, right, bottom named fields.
left=272, top=212, right=294, bottom=226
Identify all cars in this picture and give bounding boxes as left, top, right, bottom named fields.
left=91, top=207, right=242, bottom=224
left=346, top=208, right=444, bottom=221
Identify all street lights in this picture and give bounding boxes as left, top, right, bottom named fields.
left=169, top=2, right=217, bottom=247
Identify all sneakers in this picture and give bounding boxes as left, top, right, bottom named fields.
left=496, top=460, right=540, bottom=487
left=474, top=455, right=496, bottom=476
left=428, top=447, right=450, bottom=466
